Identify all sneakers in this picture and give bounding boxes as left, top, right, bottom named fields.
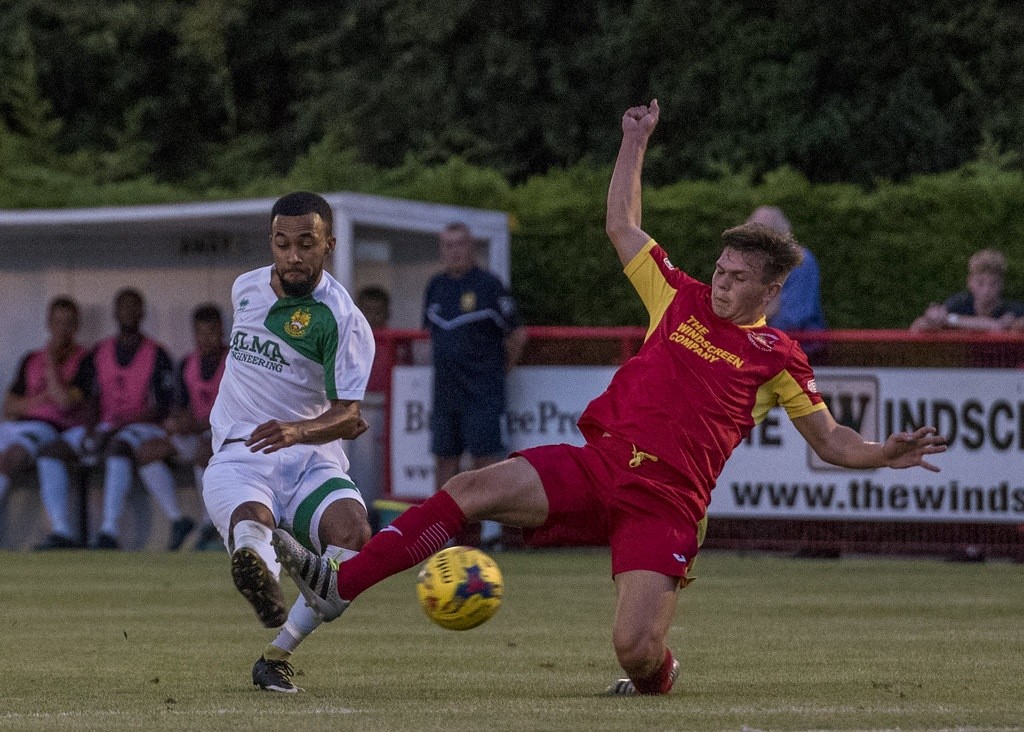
left=270, top=528, right=350, bottom=622
left=231, top=547, right=289, bottom=628
left=612, top=658, right=680, bottom=694
left=252, top=652, right=298, bottom=693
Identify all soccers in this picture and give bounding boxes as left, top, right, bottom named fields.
left=414, top=544, right=506, bottom=633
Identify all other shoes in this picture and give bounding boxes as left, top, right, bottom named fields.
left=196, top=522, right=220, bottom=550
left=167, top=516, right=197, bottom=551
left=29, top=531, right=83, bottom=550
left=95, top=533, right=119, bottom=548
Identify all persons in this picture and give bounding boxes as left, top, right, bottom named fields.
left=746, top=201, right=843, bottom=332
left=0, top=295, right=91, bottom=501
left=270, top=99, right=948, bottom=694
left=199, top=193, right=377, bottom=694
left=909, top=248, right=1024, bottom=367
left=355, top=285, right=415, bottom=393
left=31, top=288, right=172, bottom=552
left=420, top=221, right=528, bottom=548
left=137, top=303, right=229, bottom=553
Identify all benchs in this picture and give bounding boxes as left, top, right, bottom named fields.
left=84, top=471, right=224, bottom=551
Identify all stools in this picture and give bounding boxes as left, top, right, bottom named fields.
left=0, top=464, right=81, bottom=551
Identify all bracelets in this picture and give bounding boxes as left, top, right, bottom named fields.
left=947, top=313, right=957, bottom=327
